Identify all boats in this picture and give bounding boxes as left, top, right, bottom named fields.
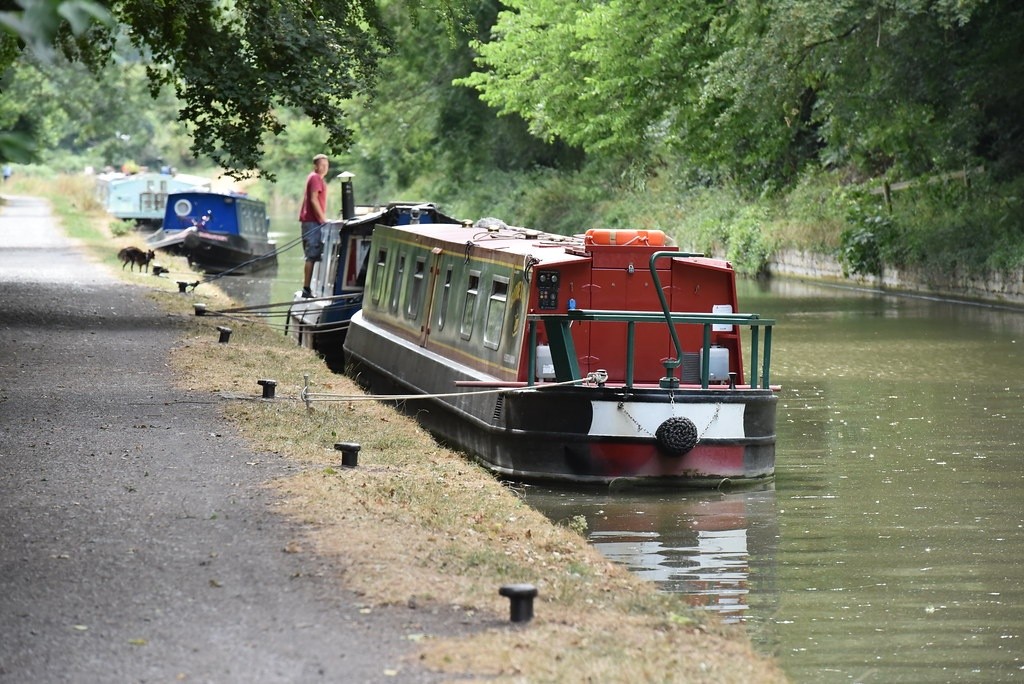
left=146, top=191, right=278, bottom=275
left=288, top=169, right=473, bottom=367
left=340, top=214, right=782, bottom=491
left=94, top=161, right=211, bottom=228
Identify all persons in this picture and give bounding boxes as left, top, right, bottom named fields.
left=3, top=164, right=11, bottom=180
left=299, top=154, right=329, bottom=298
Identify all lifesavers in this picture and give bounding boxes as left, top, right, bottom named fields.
left=585, top=230, right=668, bottom=247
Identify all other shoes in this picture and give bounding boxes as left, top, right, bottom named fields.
left=302, top=287, right=318, bottom=299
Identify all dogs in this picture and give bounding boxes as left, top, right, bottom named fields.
left=117, top=246, right=155, bottom=273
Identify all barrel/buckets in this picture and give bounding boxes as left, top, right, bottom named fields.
left=700, top=343, right=730, bottom=381
left=537, top=346, right=555, bottom=379
